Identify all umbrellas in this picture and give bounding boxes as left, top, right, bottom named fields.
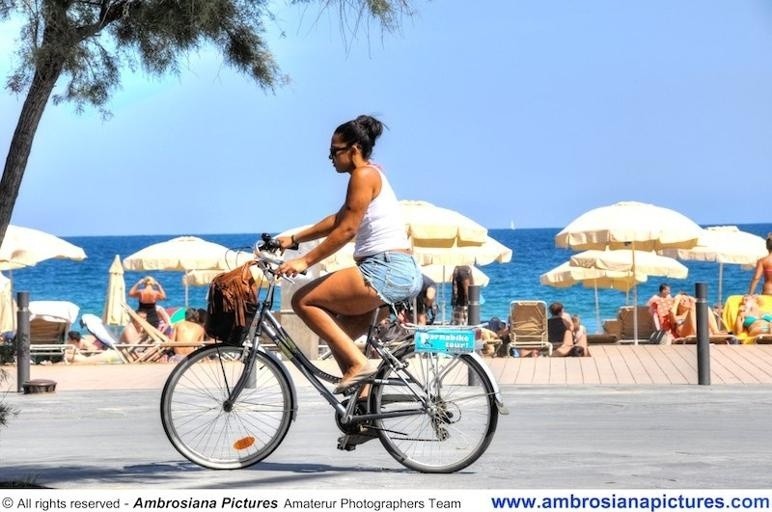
left=384, top=195, right=488, bottom=250
left=408, top=238, right=515, bottom=326
left=418, top=262, right=490, bottom=286
left=180, top=249, right=280, bottom=287
left=1, top=224, right=89, bottom=271
left=102, top=253, right=127, bottom=328
left=539, top=260, right=642, bottom=334
left=554, top=200, right=709, bottom=344
left=661, top=224, right=769, bottom=330
left=122, top=234, right=264, bottom=294
left=567, top=246, right=688, bottom=307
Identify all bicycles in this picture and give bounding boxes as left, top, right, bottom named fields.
left=159, top=233, right=510, bottom=474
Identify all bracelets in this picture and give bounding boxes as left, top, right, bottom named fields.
left=287, top=232, right=302, bottom=249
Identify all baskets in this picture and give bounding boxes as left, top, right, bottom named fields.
left=205, top=286, right=260, bottom=346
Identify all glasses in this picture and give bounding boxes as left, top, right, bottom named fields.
left=330, top=146, right=349, bottom=155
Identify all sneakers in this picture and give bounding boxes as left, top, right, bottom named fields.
left=333, top=359, right=378, bottom=394
left=338, top=429, right=377, bottom=446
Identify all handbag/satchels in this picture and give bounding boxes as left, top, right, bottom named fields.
left=649, top=330, right=672, bottom=345
left=212, top=261, right=259, bottom=327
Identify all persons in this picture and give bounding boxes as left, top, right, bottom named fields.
left=271, top=113, right=425, bottom=446
left=669, top=293, right=724, bottom=338
left=644, top=283, right=686, bottom=334
left=476, top=301, right=590, bottom=358
left=447, top=263, right=473, bottom=326
left=65, top=330, right=103, bottom=358
left=748, top=231, right=772, bottom=300
left=126, top=277, right=166, bottom=329
left=731, top=292, right=772, bottom=347
left=401, top=273, right=438, bottom=325
left=171, top=308, right=206, bottom=360
left=117, top=310, right=148, bottom=351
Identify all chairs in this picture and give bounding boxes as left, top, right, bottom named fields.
left=27, top=300, right=79, bottom=365
left=80, top=301, right=174, bottom=364
left=506, top=300, right=552, bottom=358
left=601, top=293, right=771, bottom=346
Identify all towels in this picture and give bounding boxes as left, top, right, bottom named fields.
left=28, top=301, right=78, bottom=326
